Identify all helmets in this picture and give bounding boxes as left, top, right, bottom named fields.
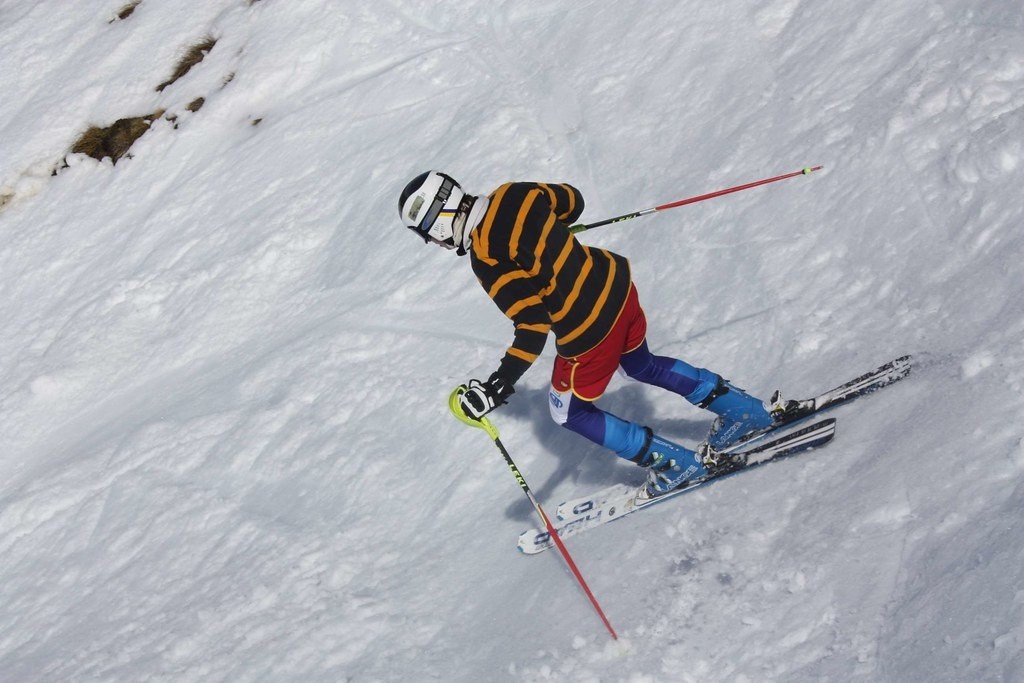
left=397, top=170, right=466, bottom=248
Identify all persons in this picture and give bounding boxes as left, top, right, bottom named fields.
left=398, top=170, right=776, bottom=497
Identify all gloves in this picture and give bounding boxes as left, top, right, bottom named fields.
left=460, top=371, right=516, bottom=421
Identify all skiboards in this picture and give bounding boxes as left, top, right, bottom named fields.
left=516, top=354, right=914, bottom=556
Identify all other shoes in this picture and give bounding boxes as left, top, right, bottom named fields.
left=709, top=406, right=776, bottom=452
left=638, top=456, right=708, bottom=500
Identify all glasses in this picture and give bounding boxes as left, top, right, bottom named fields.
left=406, top=225, right=431, bottom=244
